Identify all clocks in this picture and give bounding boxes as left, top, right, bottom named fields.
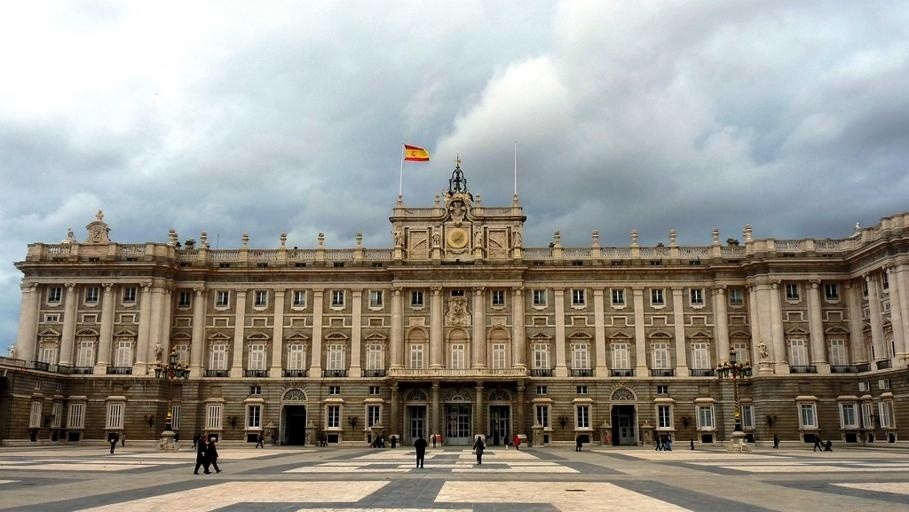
left=447, top=228, right=468, bottom=249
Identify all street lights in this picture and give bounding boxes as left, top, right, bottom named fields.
left=154, top=347, right=191, bottom=450
left=716, top=348, right=753, bottom=453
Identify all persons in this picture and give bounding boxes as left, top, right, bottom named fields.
left=503, top=434, right=509, bottom=448
left=667, top=430, right=672, bottom=450
left=320, top=430, right=326, bottom=447
left=654, top=431, right=662, bottom=451
left=256, top=433, right=263, bottom=448
left=204, top=436, right=223, bottom=473
left=325, top=430, right=328, bottom=446
left=575, top=434, right=582, bottom=452
left=375, top=433, right=381, bottom=448
left=472, top=436, right=485, bottom=465
left=414, top=433, right=427, bottom=468
left=512, top=433, right=521, bottom=450
left=381, top=432, right=385, bottom=447
left=813, top=434, right=822, bottom=451
left=771, top=433, right=780, bottom=450
left=108, top=428, right=118, bottom=453
left=193, top=437, right=212, bottom=475
left=432, top=433, right=436, bottom=448
left=659, top=434, right=668, bottom=451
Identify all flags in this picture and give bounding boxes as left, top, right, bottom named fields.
left=404, top=144, right=430, bottom=162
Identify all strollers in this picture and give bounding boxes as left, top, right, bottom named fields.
left=821, top=441, right=832, bottom=451
